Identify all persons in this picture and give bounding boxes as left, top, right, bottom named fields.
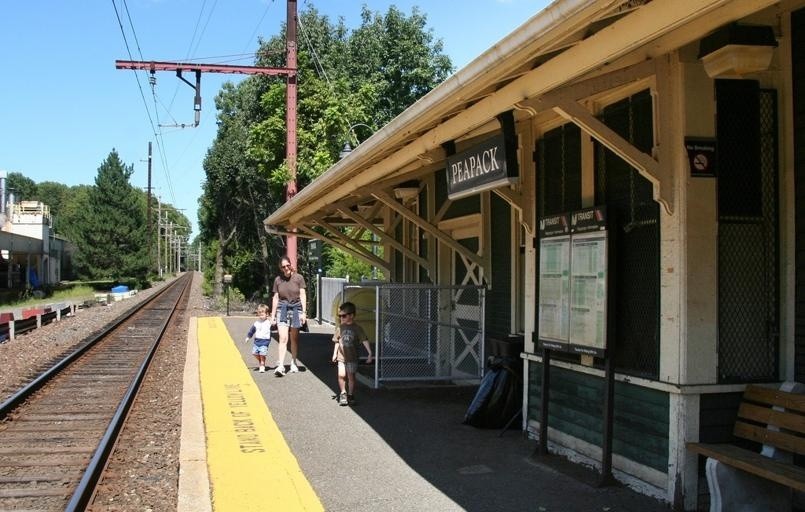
left=245, top=303, right=276, bottom=372
left=270, top=256, right=307, bottom=376
left=331, top=302, right=372, bottom=406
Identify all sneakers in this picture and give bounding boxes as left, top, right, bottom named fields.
left=259, top=366, right=265, bottom=373
left=339, top=392, right=348, bottom=405
left=290, top=362, right=298, bottom=373
left=347, top=394, right=354, bottom=404
left=274, top=365, right=285, bottom=376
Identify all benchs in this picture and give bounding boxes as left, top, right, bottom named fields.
left=682, top=379, right=805, bottom=511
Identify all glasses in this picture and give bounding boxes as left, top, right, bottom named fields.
left=339, top=313, right=352, bottom=318
